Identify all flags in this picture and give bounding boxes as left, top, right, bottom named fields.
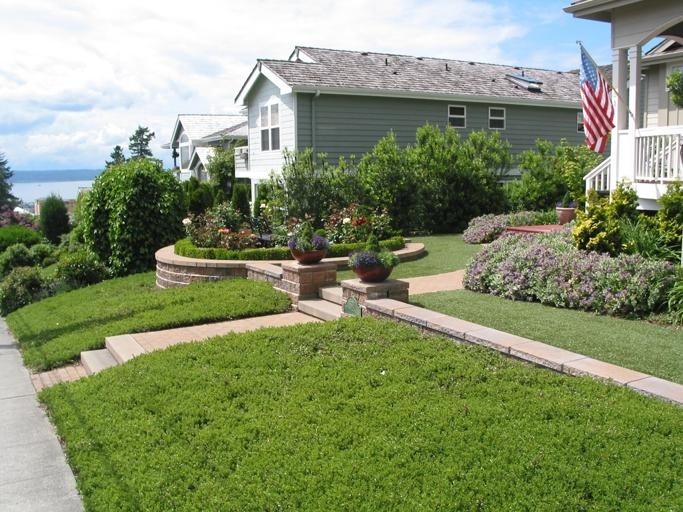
left=574, top=40, right=615, bottom=156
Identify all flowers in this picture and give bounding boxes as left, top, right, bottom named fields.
left=286, top=219, right=330, bottom=251
left=346, top=229, right=399, bottom=271
left=555, top=191, right=577, bottom=208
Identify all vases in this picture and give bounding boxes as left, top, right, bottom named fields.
left=555, top=207, right=577, bottom=225
left=288, top=249, right=328, bottom=265
left=350, top=264, right=393, bottom=283
